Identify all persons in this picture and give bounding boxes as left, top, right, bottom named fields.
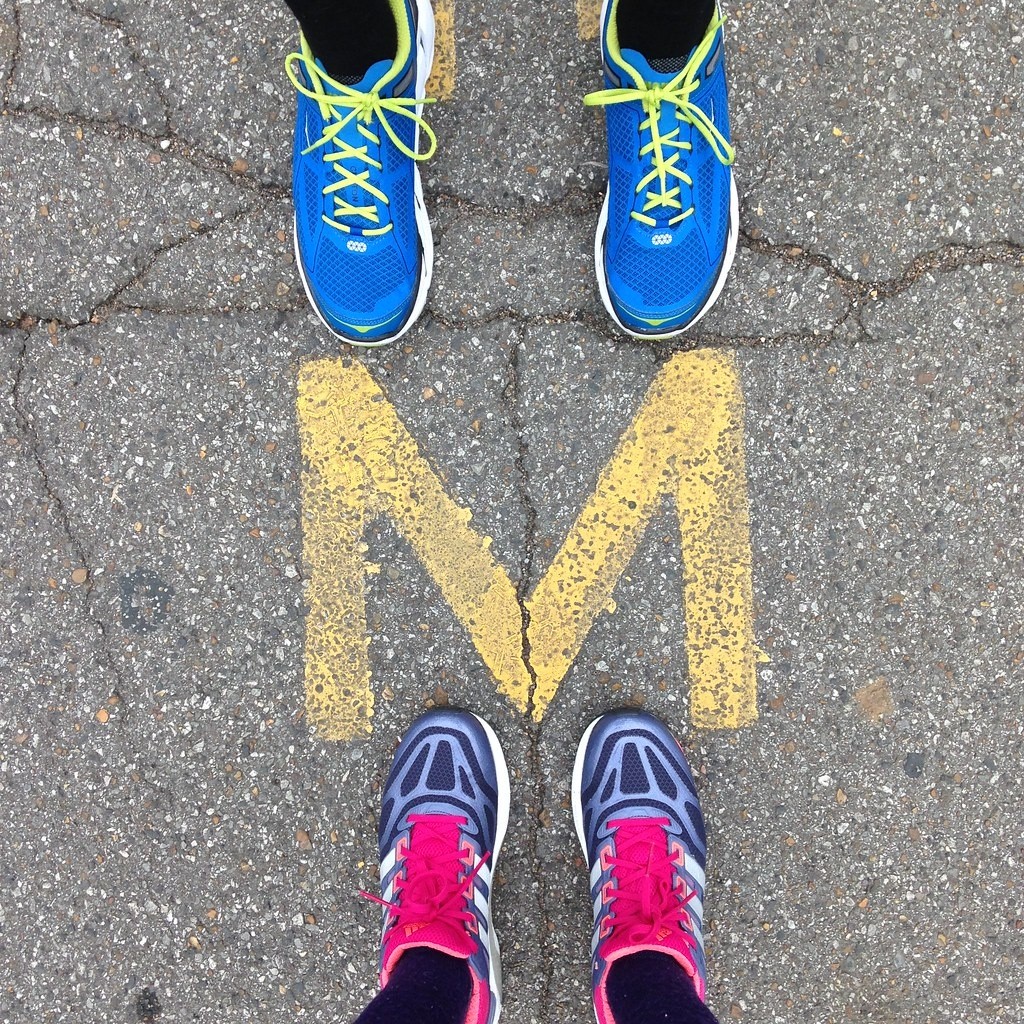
left=347, top=710, right=729, bottom=1024
left=282, top=1, right=741, bottom=348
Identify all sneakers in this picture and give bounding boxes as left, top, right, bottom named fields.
left=284, top=0, right=438, bottom=348
left=583, top=0, right=740, bottom=342
left=359, top=705, right=511, bottom=1024
left=570, top=707, right=707, bottom=1024
